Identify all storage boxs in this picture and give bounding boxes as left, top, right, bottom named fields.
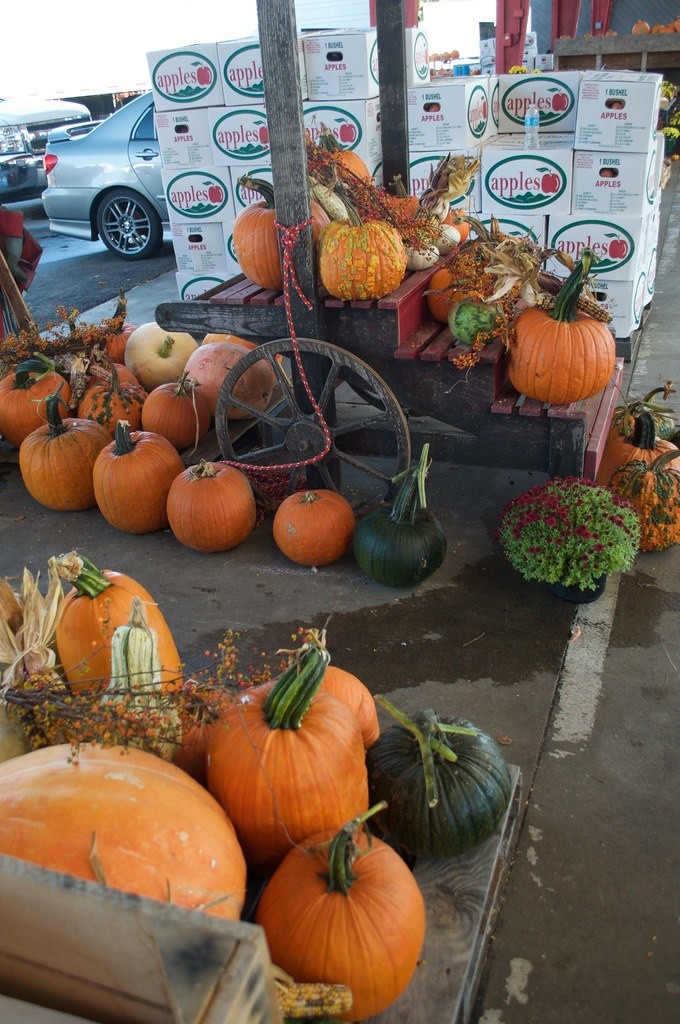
left=408, top=26, right=665, bottom=338
left=146, top=25, right=382, bottom=300
left=0, top=855, right=284, bottom=1024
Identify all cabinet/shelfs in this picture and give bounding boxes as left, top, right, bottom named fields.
left=155, top=240, right=624, bottom=480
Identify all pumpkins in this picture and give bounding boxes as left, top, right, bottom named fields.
left=595, top=384, right=680, bottom=553
left=0, top=290, right=449, bottom=588
left=233, top=132, right=615, bottom=406
left=0, top=550, right=511, bottom=1024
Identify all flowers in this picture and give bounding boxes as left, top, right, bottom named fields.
left=498, top=476, right=640, bottom=574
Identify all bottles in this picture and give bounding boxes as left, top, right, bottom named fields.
left=523, top=104, right=540, bottom=149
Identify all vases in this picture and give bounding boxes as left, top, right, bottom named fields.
left=547, top=575, right=605, bottom=605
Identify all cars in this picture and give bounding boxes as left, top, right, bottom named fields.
left=40, top=91, right=170, bottom=262
left=0, top=99, right=95, bottom=208
